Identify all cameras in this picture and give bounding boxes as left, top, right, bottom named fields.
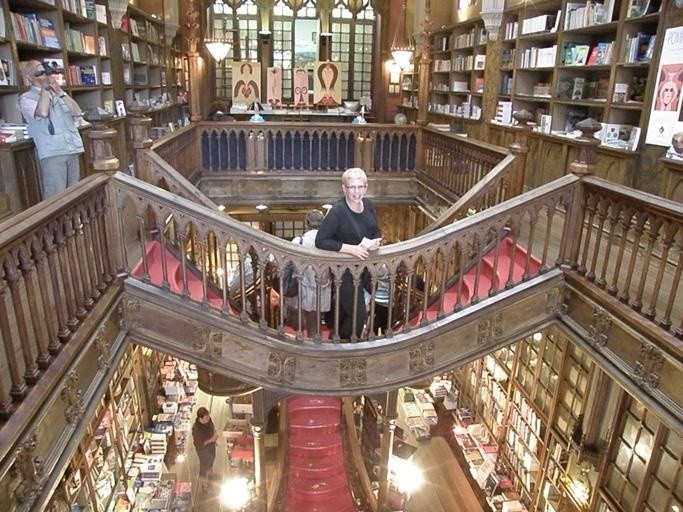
left=42, top=61, right=65, bottom=75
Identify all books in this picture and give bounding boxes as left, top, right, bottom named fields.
left=398, top=354, right=609, bottom=511
left=0, top=0, right=191, bottom=142
left=45, top=344, right=198, bottom=512
left=400, top=0, right=663, bottom=149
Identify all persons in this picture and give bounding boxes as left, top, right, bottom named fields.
left=192, top=406, right=219, bottom=494
left=267, top=402, right=280, bottom=460
left=287, top=209, right=332, bottom=340
left=355, top=394, right=381, bottom=454
left=314, top=167, right=383, bottom=343
left=18, top=59, right=84, bottom=200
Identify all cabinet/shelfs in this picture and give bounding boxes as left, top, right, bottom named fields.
left=452, top=353, right=620, bottom=512
left=426, top=12, right=500, bottom=144
left=397, top=73, right=418, bottom=126
left=0, top=0, right=124, bottom=221
left=221, top=397, right=253, bottom=472
left=494, top=0, right=683, bottom=194
left=39, top=346, right=165, bottom=512
left=122, top=4, right=186, bottom=172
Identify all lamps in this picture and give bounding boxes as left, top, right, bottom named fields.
left=390, top=2, right=415, bottom=72
left=204, top=0, right=235, bottom=65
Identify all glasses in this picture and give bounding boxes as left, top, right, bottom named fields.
left=346, top=185, right=365, bottom=190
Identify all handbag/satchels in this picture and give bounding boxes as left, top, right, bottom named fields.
left=274, top=263, right=297, bottom=296
